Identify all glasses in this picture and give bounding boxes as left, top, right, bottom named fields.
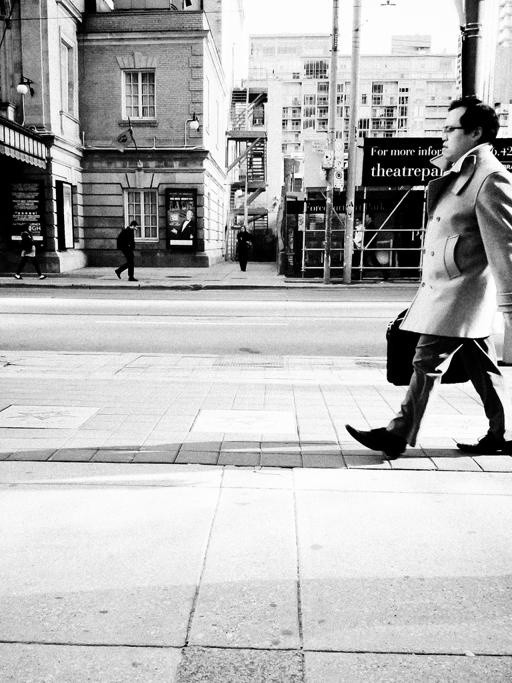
left=442, top=126, right=463, bottom=132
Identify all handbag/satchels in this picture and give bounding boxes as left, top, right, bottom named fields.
left=386, top=307, right=489, bottom=386
left=20, top=230, right=36, bottom=258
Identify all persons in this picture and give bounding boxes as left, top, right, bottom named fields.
left=346, top=93, right=511, bottom=458
left=234, top=225, right=251, bottom=270
left=114, top=219, right=139, bottom=280
left=355, top=213, right=389, bottom=280
left=350, top=217, right=364, bottom=280
left=170, top=207, right=194, bottom=238
left=12, top=221, right=48, bottom=280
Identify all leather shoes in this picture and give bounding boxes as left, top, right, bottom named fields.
left=115, top=270, right=121, bottom=279
left=457, top=431, right=512, bottom=457
left=40, top=274, right=47, bottom=280
left=15, top=275, right=22, bottom=279
left=128, top=277, right=138, bottom=281
left=345, top=425, right=407, bottom=460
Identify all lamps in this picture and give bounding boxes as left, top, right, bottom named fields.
left=17, top=74, right=35, bottom=97
left=187, top=113, right=200, bottom=132
